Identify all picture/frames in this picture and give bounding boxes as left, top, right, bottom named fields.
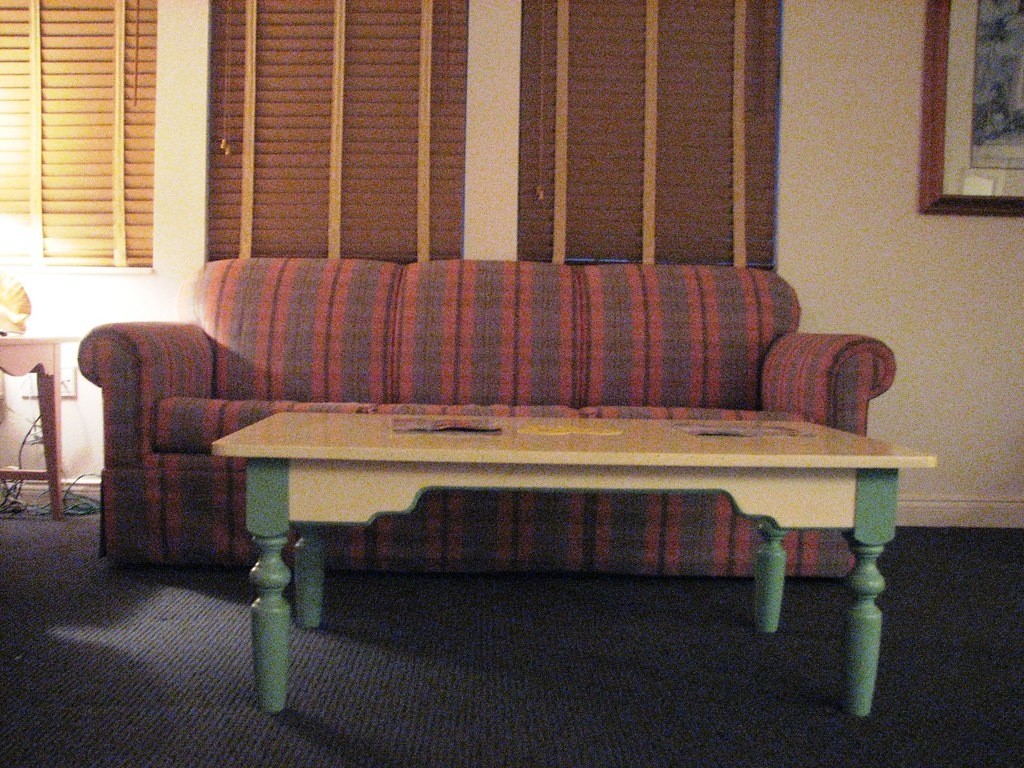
left=917, top=0, right=1024, bottom=216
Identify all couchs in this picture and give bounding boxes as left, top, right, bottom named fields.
left=78, top=258, right=896, bottom=579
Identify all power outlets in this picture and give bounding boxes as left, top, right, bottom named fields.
left=58, top=366, right=78, bottom=398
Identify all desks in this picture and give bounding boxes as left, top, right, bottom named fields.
left=0, top=336, right=81, bottom=521
left=209, top=410, right=939, bottom=717
